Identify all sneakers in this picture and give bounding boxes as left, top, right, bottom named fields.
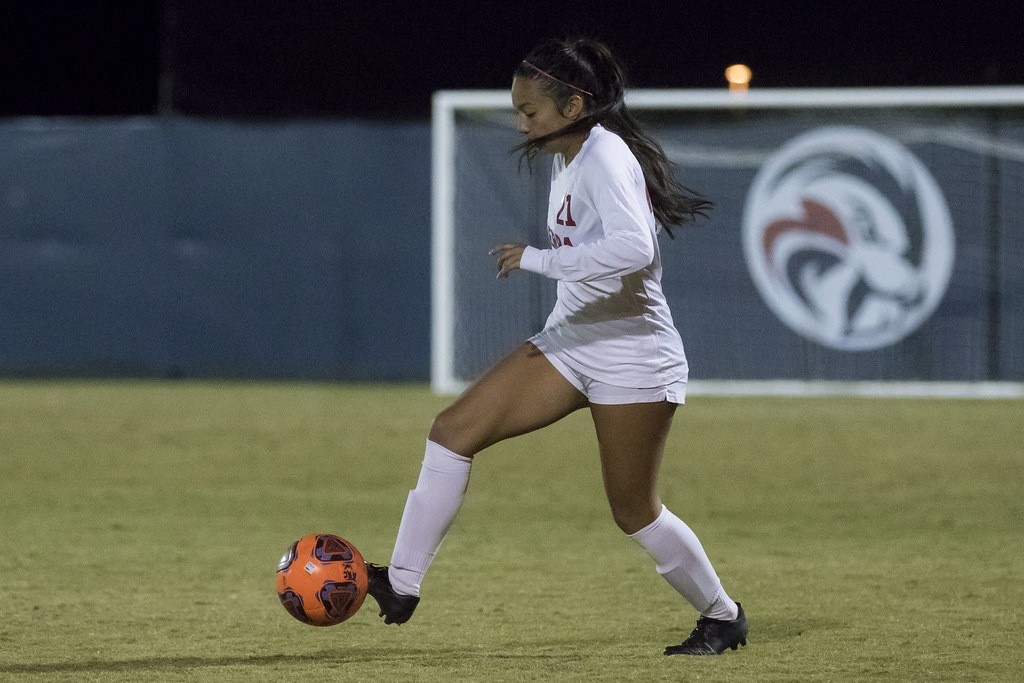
left=664, top=601, right=748, bottom=656
left=365, top=566, right=418, bottom=624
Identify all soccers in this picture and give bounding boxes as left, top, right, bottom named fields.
left=273, top=531, right=370, bottom=627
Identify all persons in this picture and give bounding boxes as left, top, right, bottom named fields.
left=366, top=37, right=748, bottom=655
